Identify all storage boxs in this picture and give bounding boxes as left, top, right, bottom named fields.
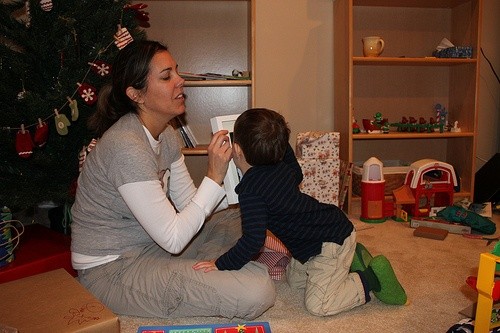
left=0, top=274, right=121, bottom=333
left=0, top=223, right=78, bottom=285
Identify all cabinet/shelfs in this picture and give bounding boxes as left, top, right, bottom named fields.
left=129, top=0, right=256, bottom=188
left=333, top=0, right=484, bottom=217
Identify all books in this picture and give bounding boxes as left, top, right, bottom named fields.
left=168, top=115, right=199, bottom=149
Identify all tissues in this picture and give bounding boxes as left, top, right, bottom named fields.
left=432, top=37, right=473, bottom=58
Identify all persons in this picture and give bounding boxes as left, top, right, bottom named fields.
left=70, top=38, right=277, bottom=321
left=192, top=108, right=407, bottom=317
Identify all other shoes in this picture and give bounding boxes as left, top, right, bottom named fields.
left=350, top=243, right=374, bottom=272
left=369, top=255, right=407, bottom=306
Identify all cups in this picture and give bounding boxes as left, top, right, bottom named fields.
left=363, top=36, right=385, bottom=57
